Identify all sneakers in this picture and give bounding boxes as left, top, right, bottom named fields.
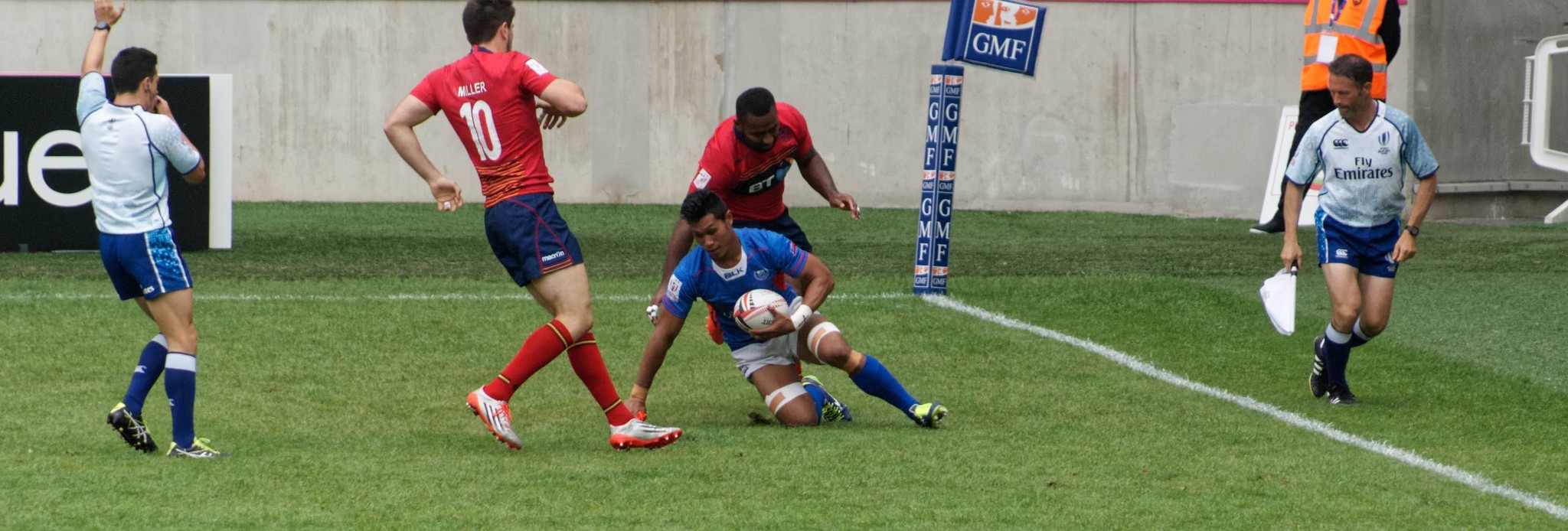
left=801, top=375, right=853, bottom=422
left=609, top=412, right=683, bottom=451
left=1309, top=334, right=1329, bottom=399
left=466, top=385, right=521, bottom=450
left=167, top=436, right=229, bottom=457
left=705, top=303, right=724, bottom=345
left=107, top=403, right=159, bottom=453
left=1325, top=382, right=1358, bottom=405
left=913, top=402, right=949, bottom=427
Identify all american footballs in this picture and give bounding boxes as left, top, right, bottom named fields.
left=733, top=289, right=790, bottom=333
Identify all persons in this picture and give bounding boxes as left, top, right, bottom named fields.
left=623, top=189, right=948, bottom=429
left=382, top=0, right=680, bottom=451
left=1281, top=54, right=1439, bottom=404
left=1250, top=0, right=1401, bottom=234
left=645, top=87, right=861, bottom=383
left=76, top=0, right=219, bottom=459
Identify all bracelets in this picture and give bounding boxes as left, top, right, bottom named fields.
left=791, top=304, right=812, bottom=331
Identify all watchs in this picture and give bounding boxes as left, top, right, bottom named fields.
left=93, top=21, right=110, bottom=32
left=1404, top=225, right=1419, bottom=236
